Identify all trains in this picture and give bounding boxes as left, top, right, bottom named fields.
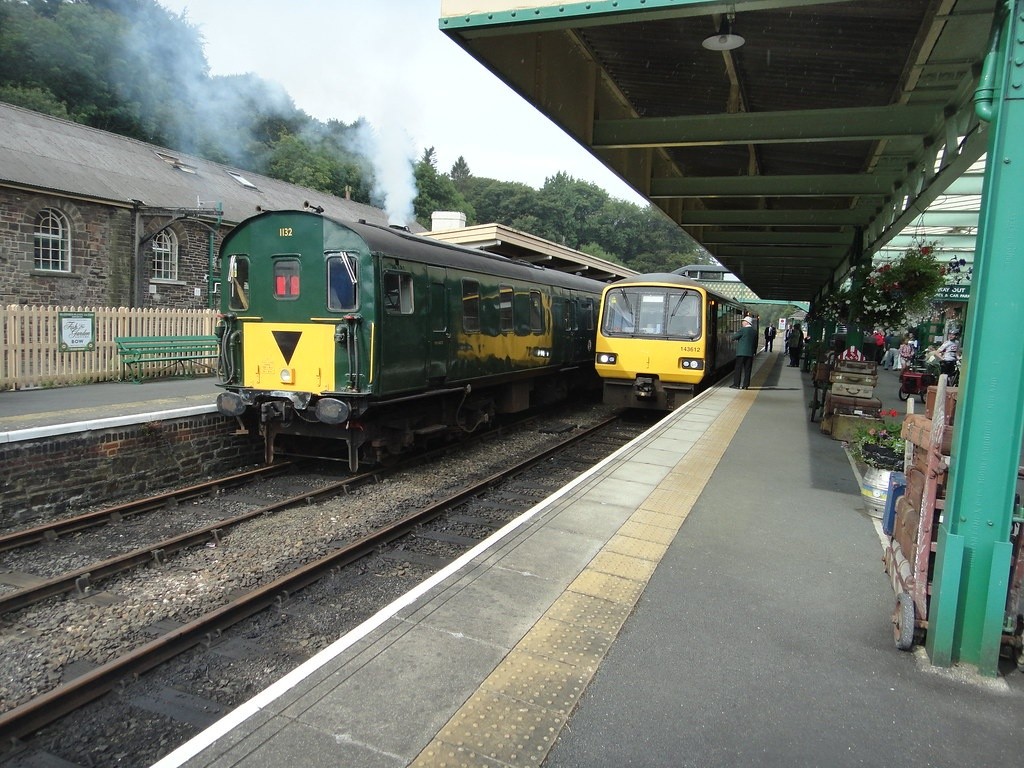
left=214, top=202, right=613, bottom=472
left=595, top=272, right=760, bottom=415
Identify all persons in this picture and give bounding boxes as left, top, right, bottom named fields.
left=872, top=319, right=961, bottom=383
left=730, top=316, right=759, bottom=389
left=764, top=323, right=777, bottom=352
left=783, top=324, right=804, bottom=368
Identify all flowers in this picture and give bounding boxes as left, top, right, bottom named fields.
left=800, top=239, right=973, bottom=326
left=838, top=407, right=905, bottom=463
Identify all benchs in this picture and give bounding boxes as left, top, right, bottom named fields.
left=113, top=334, right=219, bottom=384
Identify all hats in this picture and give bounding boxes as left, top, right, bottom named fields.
left=949, top=329, right=959, bottom=334
left=741, top=316, right=752, bottom=324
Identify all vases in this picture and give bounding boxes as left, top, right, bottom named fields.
left=861, top=441, right=904, bottom=471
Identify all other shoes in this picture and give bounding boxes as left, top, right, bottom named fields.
left=795, top=365, right=799, bottom=367
left=743, top=385, right=748, bottom=389
left=787, top=364, right=796, bottom=367
left=729, top=384, right=740, bottom=389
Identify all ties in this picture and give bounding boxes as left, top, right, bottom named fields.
left=789, top=332, right=790, bottom=336
left=770, top=329, right=771, bottom=335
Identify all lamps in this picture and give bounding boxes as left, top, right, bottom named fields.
left=702, top=12, right=746, bottom=52
